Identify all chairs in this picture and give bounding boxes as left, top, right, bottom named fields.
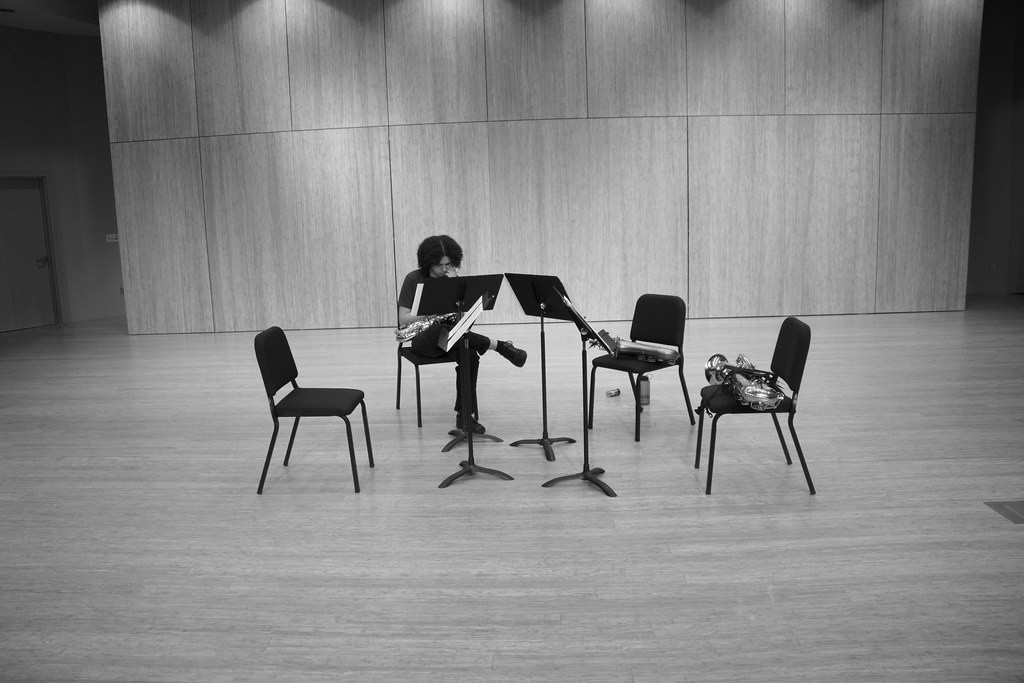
left=254, top=326, right=375, bottom=495
left=397, top=302, right=478, bottom=426
left=589, top=294, right=696, bottom=441
left=695, top=316, right=816, bottom=495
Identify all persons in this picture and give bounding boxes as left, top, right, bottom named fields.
left=398, top=235, right=527, bottom=435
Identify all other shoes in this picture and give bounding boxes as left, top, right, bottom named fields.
left=457, top=413, right=485, bottom=434
left=496, top=339, right=527, bottom=368
